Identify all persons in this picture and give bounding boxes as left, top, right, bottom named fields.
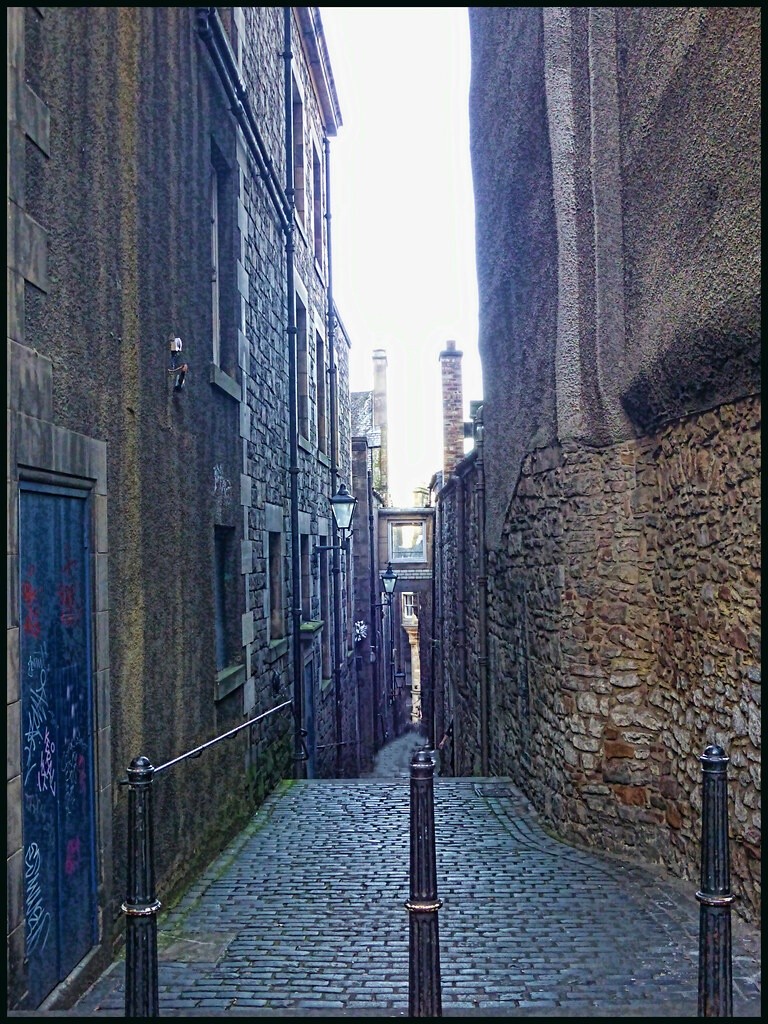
left=439, top=719, right=454, bottom=776
left=411, top=539, right=423, bottom=557
left=410, top=705, right=420, bottom=718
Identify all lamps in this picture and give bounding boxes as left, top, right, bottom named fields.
left=314, top=484, right=358, bottom=550
left=373, top=562, right=398, bottom=607
left=388, top=664, right=406, bottom=704
